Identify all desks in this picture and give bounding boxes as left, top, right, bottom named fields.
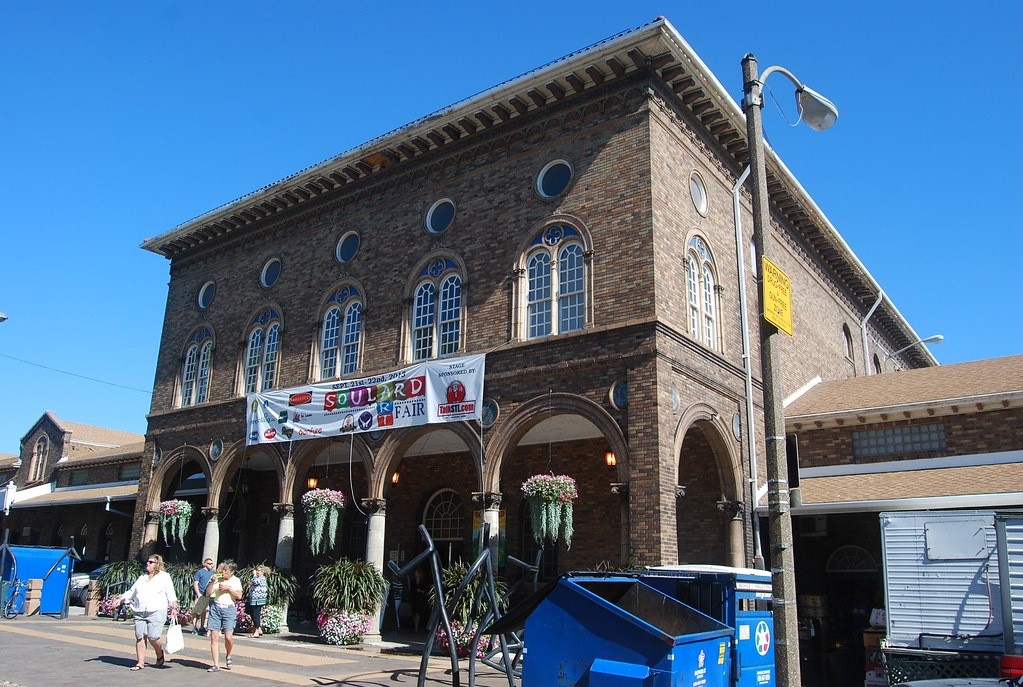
left=863, top=625, right=886, bottom=661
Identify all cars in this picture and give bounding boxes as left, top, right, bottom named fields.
left=71, top=560, right=121, bottom=606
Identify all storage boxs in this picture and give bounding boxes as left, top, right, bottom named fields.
left=863, top=664, right=888, bottom=687
left=798, top=592, right=848, bottom=619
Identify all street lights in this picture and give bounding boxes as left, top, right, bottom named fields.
left=739, top=50, right=839, bottom=687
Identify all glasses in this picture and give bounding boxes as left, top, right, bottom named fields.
left=206, top=563, right=213, bottom=565
left=148, top=561, right=156, bottom=564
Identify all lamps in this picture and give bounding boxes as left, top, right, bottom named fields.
left=604, top=445, right=617, bottom=472
left=240, top=478, right=249, bottom=497
left=391, top=466, right=399, bottom=488
left=885, top=335, right=945, bottom=361
left=306, top=459, right=318, bottom=490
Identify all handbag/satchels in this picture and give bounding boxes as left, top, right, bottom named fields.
left=166, top=616, right=184, bottom=654
left=194, top=595, right=210, bottom=616
left=235, top=586, right=254, bottom=633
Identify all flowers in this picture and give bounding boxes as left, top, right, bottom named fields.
left=232, top=558, right=301, bottom=635
left=427, top=555, right=509, bottom=660
left=309, top=557, right=391, bottom=646
left=301, top=488, right=348, bottom=557
left=92, top=560, right=146, bottom=619
left=166, top=561, right=203, bottom=627
left=157, top=498, right=193, bottom=551
left=520, top=471, right=579, bottom=551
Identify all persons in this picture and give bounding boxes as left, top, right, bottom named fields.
left=206, top=561, right=243, bottom=672
left=390, top=573, right=403, bottom=628
left=112, top=554, right=179, bottom=671
left=192, top=558, right=225, bottom=637
left=407, top=568, right=425, bottom=632
left=300, top=597, right=317, bottom=624
left=248, top=565, right=268, bottom=638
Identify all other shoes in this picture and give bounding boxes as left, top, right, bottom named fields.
left=191, top=627, right=200, bottom=636
left=199, top=627, right=207, bottom=635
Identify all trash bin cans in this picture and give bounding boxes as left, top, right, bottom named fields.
left=629, top=565, right=776, bottom=687
left=480, top=571, right=734, bottom=687
left=0, top=544, right=68, bottom=615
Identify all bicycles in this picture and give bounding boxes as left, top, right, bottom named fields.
left=4, top=578, right=31, bottom=619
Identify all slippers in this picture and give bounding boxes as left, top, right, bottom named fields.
left=226, top=656, right=233, bottom=669
left=207, top=665, right=220, bottom=672
left=130, top=666, right=142, bottom=671
left=156, top=650, right=165, bottom=666
left=259, top=632, right=263, bottom=636
left=248, top=635, right=259, bottom=638
